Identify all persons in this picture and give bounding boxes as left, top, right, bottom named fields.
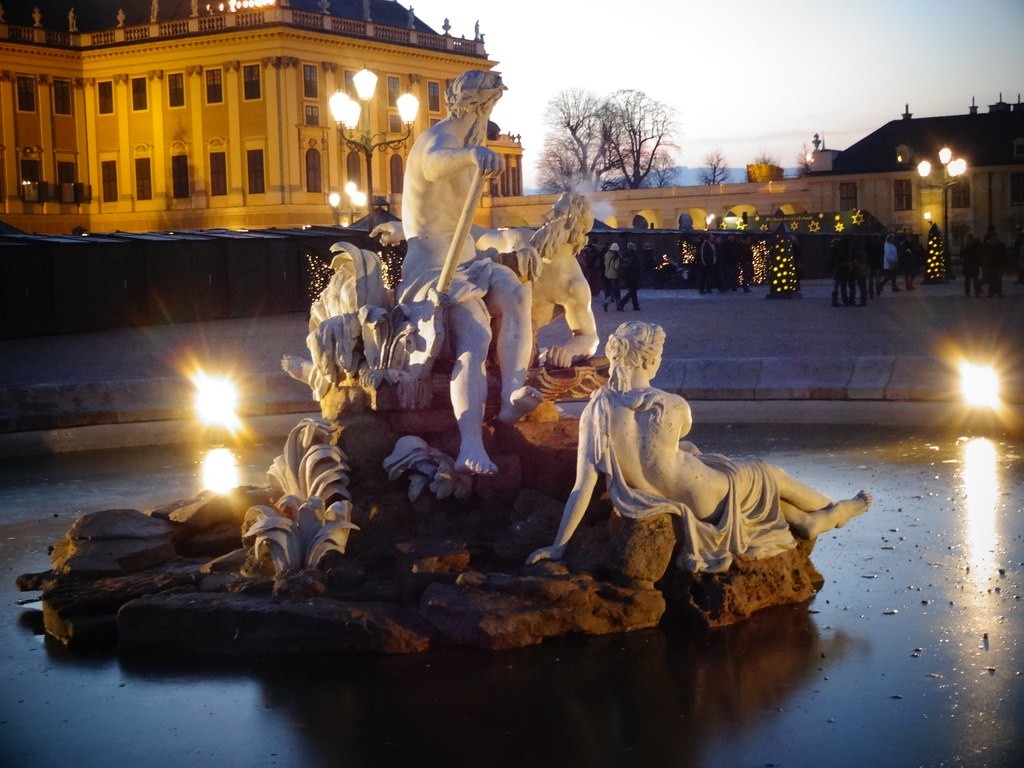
left=616, top=242, right=641, bottom=313
left=601, top=242, right=623, bottom=313
left=698, top=232, right=755, bottom=294
left=525, top=320, right=876, bottom=575
left=395, top=68, right=546, bottom=477
left=575, top=234, right=615, bottom=303
left=827, top=232, right=921, bottom=309
left=279, top=190, right=601, bottom=387
left=960, top=224, right=1024, bottom=300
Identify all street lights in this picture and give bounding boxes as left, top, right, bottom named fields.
left=919, top=145, right=968, bottom=278
left=328, top=181, right=367, bottom=227
left=329, top=69, right=419, bottom=236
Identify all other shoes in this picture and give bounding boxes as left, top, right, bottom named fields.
left=604, top=303, right=608, bottom=312
left=892, top=286, right=901, bottom=292
left=619, top=302, right=623, bottom=310
left=879, top=282, right=885, bottom=291
left=634, top=308, right=640, bottom=310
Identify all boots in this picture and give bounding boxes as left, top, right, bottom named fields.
left=832, top=291, right=841, bottom=307
left=841, top=289, right=848, bottom=304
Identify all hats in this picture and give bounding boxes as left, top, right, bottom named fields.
left=627, top=242, right=636, bottom=250
left=610, top=243, right=619, bottom=251
